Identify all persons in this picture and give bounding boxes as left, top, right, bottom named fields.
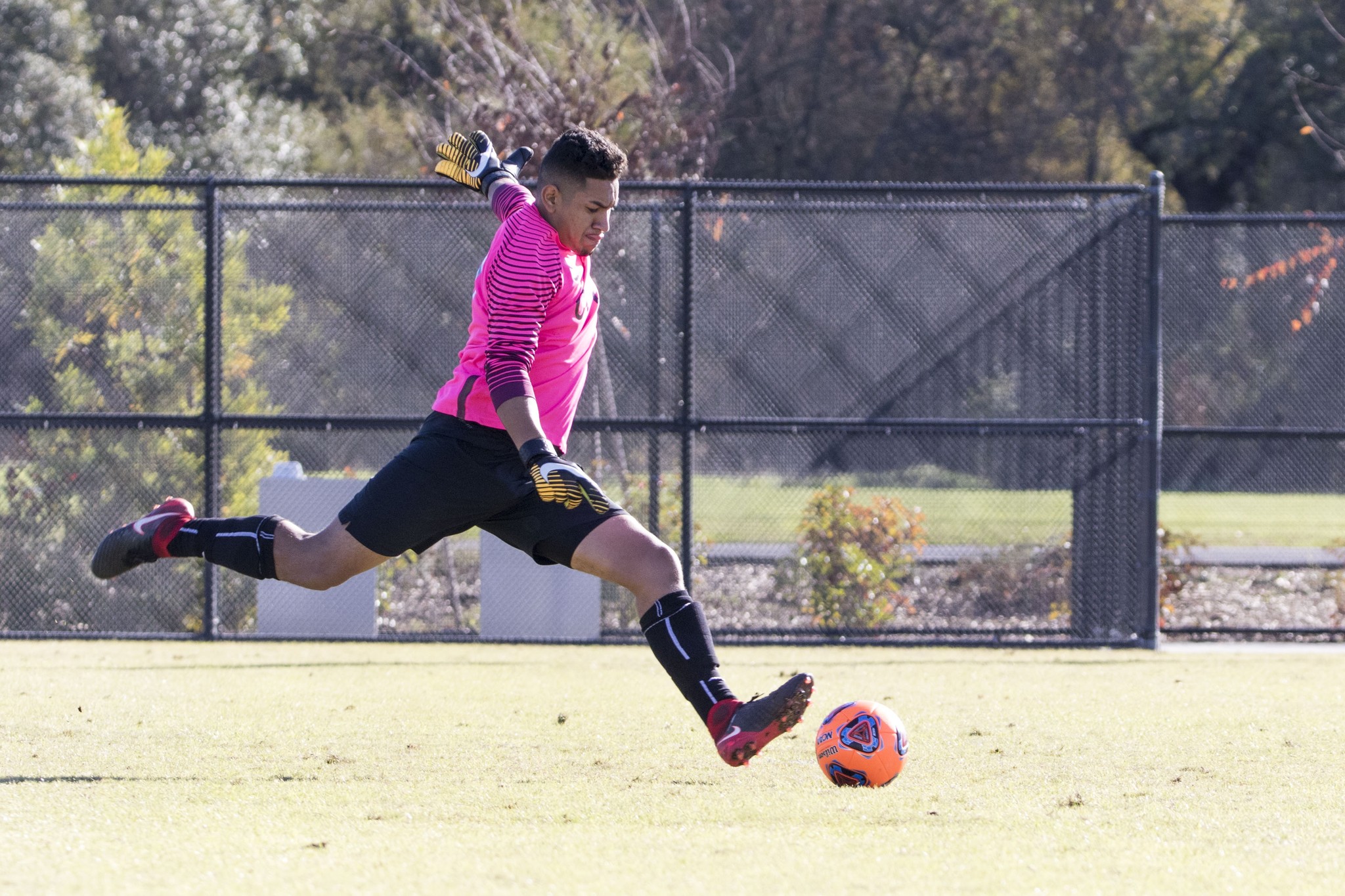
left=89, top=124, right=815, bottom=767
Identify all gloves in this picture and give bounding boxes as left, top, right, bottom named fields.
left=434, top=129, right=534, bottom=200
left=518, top=437, right=611, bottom=516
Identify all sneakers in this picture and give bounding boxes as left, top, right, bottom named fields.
left=716, top=671, right=817, bottom=768
left=90, top=496, right=196, bottom=580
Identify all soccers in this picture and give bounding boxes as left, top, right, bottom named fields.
left=818, top=699, right=905, bottom=787
